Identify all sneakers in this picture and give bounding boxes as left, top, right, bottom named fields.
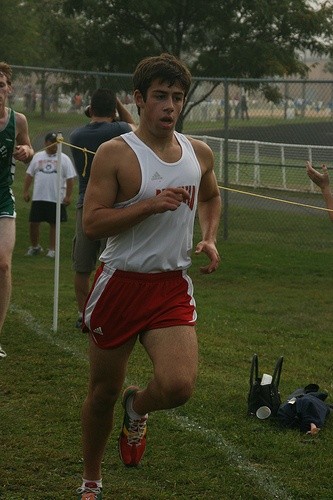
left=46, top=248, right=55, bottom=257
left=119, top=386, right=149, bottom=467
left=77, top=479, right=103, bottom=500
left=0, top=344, right=7, bottom=357
left=77, top=313, right=84, bottom=331
left=24, top=245, right=43, bottom=257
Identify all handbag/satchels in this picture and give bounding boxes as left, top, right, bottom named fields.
left=247, top=354, right=284, bottom=415
left=278, top=383, right=329, bottom=432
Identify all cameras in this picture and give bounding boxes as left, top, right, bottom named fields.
left=85, top=105, right=91, bottom=118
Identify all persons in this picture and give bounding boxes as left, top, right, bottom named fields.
left=69, top=88, right=138, bottom=327
left=23, top=133, right=77, bottom=258
left=307, top=161, right=333, bottom=223
left=0, top=60, right=35, bottom=357
left=76, top=53, right=222, bottom=500
left=7, top=78, right=324, bottom=121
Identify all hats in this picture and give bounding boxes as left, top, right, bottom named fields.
left=46, top=133, right=56, bottom=142
